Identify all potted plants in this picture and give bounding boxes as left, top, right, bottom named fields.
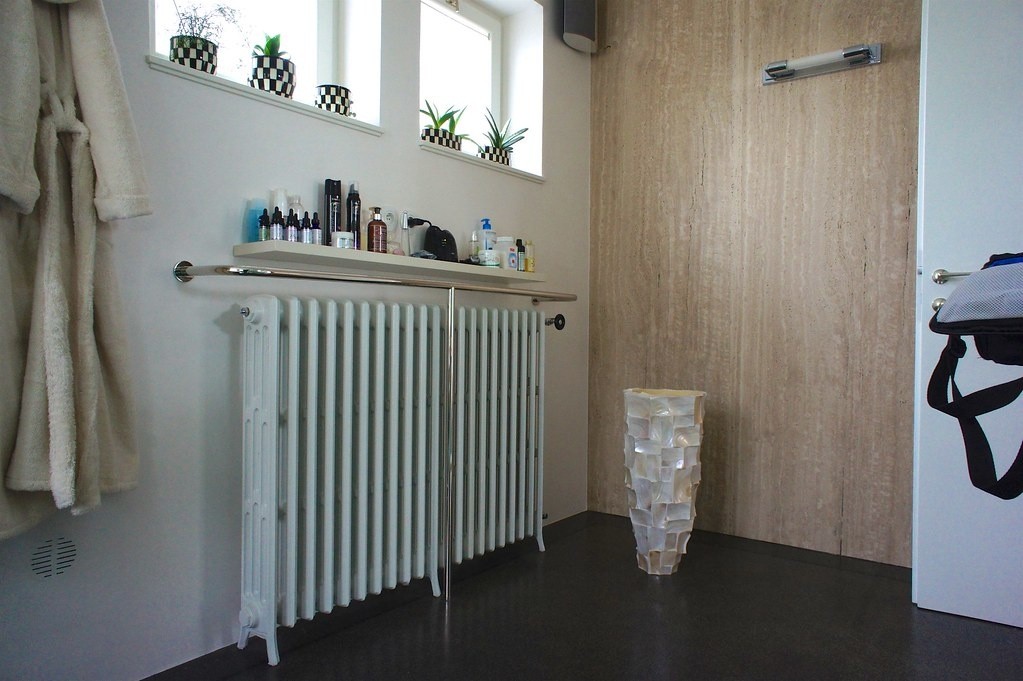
left=168, top=0, right=241, bottom=76
left=246, top=32, right=299, bottom=99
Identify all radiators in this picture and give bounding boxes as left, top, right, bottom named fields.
left=238, top=292, right=551, bottom=667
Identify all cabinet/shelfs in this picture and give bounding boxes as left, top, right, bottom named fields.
left=232, top=237, right=550, bottom=286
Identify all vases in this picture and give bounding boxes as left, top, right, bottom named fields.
left=419, top=99, right=487, bottom=156
left=314, top=83, right=354, bottom=118
left=475, top=107, right=530, bottom=167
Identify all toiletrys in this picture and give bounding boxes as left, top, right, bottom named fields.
left=244, top=179, right=536, bottom=273
